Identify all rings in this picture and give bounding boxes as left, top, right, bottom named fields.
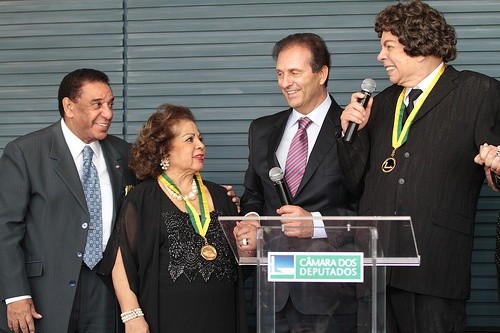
left=242, top=238, right=248, bottom=246
left=496, top=151, right=500, bottom=157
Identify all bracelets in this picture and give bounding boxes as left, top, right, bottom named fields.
left=121, top=307, right=145, bottom=324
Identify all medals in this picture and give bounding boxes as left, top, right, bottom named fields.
left=201, top=244, right=217, bottom=260
left=382, top=158, right=396, bottom=173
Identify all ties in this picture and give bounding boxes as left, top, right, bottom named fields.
left=284, top=116, right=312, bottom=197
left=401, top=88, right=423, bottom=130
left=81, top=145, right=103, bottom=269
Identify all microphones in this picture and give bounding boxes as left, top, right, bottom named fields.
left=345, top=78, right=377, bottom=143
left=269, top=167, right=293, bottom=206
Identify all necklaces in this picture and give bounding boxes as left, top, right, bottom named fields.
left=164, top=177, right=199, bottom=202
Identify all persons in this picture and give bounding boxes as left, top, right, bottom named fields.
left=339, top=0, right=500, bottom=333
left=0, top=67, right=257, bottom=333
left=232, top=32, right=369, bottom=333
